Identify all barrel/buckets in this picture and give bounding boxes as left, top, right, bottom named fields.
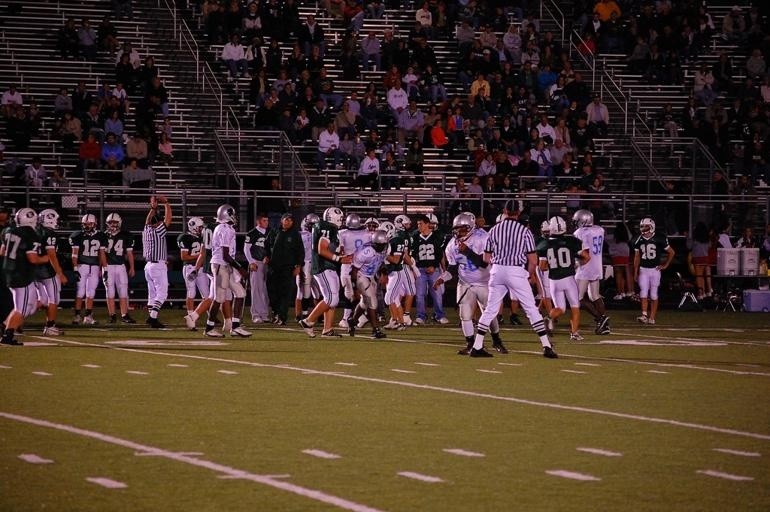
left=740, top=247, right=761, bottom=276
left=716, top=246, right=740, bottom=277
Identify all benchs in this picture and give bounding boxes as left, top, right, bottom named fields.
left=0, top=2, right=770, bottom=224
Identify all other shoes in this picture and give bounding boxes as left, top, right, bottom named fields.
left=301, top=314, right=310, bottom=319
left=273, top=314, right=286, bottom=326
left=253, top=318, right=263, bottom=324
left=262, top=317, right=270, bottom=322
left=295, top=315, right=302, bottom=321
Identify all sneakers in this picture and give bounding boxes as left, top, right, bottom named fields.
left=511, top=313, right=523, bottom=326
left=321, top=328, right=343, bottom=338
left=298, top=319, right=316, bottom=337
left=613, top=288, right=714, bottom=324
left=184, top=314, right=198, bottom=331
left=145, top=315, right=168, bottom=330
left=119, top=313, right=136, bottom=324
left=43, top=325, right=64, bottom=336
left=339, top=310, right=448, bottom=330
left=106, top=314, right=117, bottom=325
left=595, top=313, right=610, bottom=335
left=543, top=342, right=558, bottom=356
left=1, top=336, right=24, bottom=346
left=229, top=327, right=253, bottom=337
left=203, top=328, right=225, bottom=338
left=469, top=347, right=493, bottom=357
left=497, top=315, right=503, bottom=324
left=82, top=314, right=97, bottom=325
left=570, top=332, right=585, bottom=340
left=544, top=316, right=553, bottom=332
left=492, top=342, right=508, bottom=354
left=222, top=327, right=232, bottom=333
left=601, top=325, right=609, bottom=335
left=71, top=314, right=82, bottom=325
left=457, top=345, right=471, bottom=355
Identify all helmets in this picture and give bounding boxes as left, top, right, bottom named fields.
left=15, top=204, right=235, bottom=234
left=640, top=218, right=656, bottom=234
left=301, top=207, right=593, bottom=244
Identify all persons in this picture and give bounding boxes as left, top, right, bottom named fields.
left=0, top=0, right=770, bottom=345
left=433, top=201, right=561, bottom=358
